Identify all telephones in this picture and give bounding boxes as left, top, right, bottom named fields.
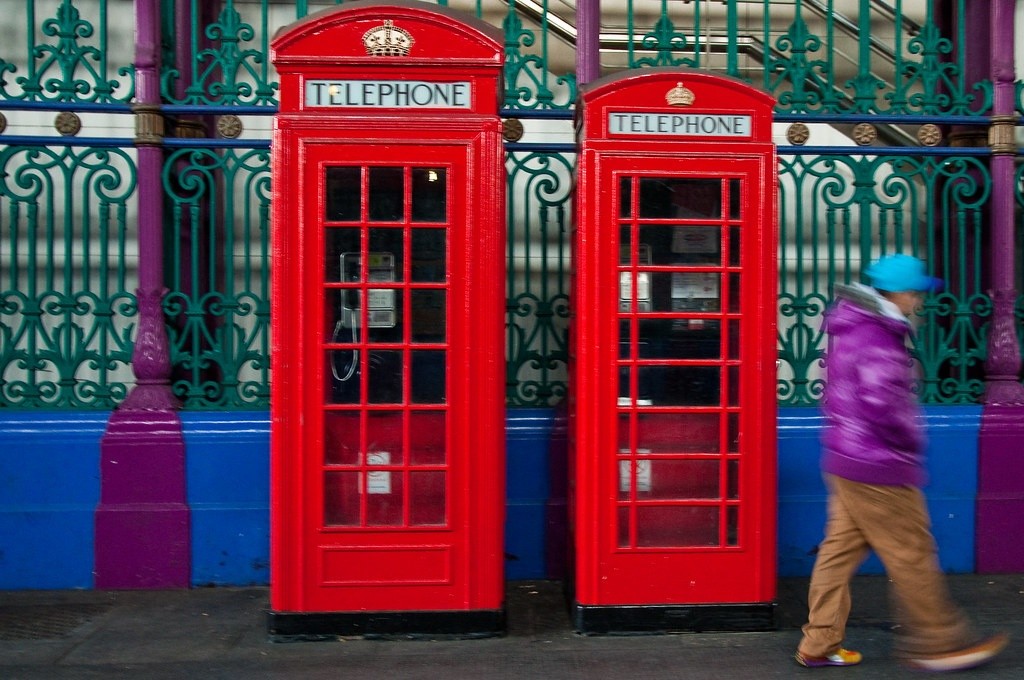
left=339, top=250, right=398, bottom=329
left=621, top=242, right=652, bottom=314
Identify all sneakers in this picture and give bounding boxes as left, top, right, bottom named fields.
left=793, top=646, right=864, bottom=667
left=910, top=634, right=1010, bottom=672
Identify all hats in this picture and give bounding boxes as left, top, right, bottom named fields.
left=860, top=253, right=946, bottom=294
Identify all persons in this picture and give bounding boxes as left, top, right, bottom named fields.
left=795, top=255, right=1008, bottom=669
